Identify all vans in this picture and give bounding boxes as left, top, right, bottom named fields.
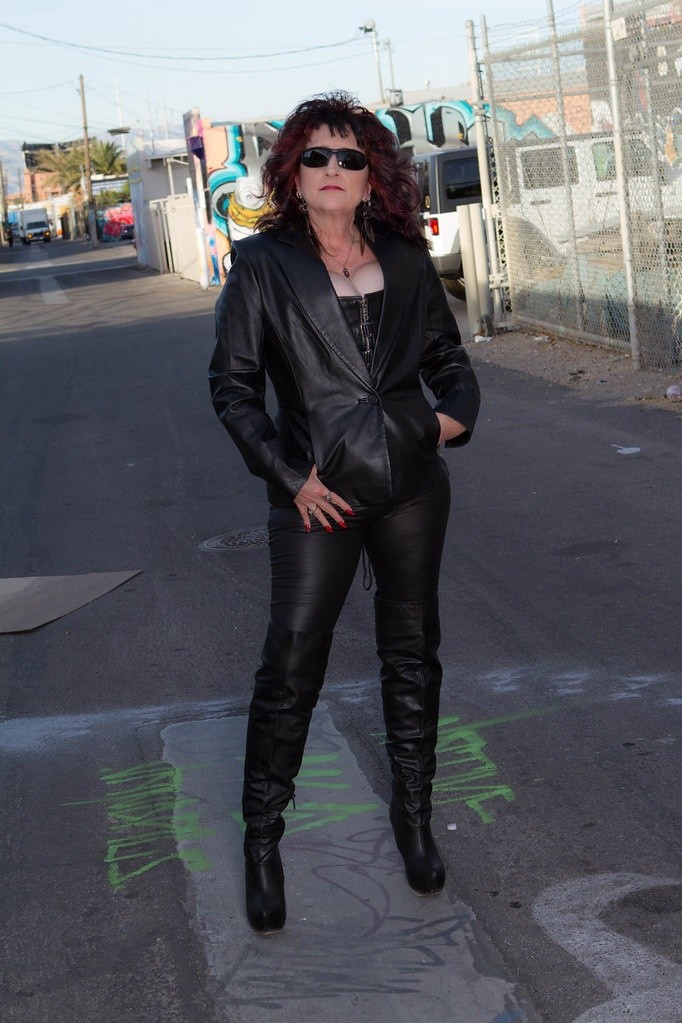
left=408, top=130, right=661, bottom=311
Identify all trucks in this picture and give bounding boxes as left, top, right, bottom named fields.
left=17, top=208, right=53, bottom=246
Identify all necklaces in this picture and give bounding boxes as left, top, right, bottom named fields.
left=318, top=226, right=355, bottom=277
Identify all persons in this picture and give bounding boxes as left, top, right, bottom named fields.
left=6, top=228, right=13, bottom=248
left=208, top=90, right=481, bottom=934
left=606, top=143, right=616, bottom=179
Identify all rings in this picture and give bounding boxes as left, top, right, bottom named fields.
left=323, top=491, right=332, bottom=503
left=309, top=505, right=317, bottom=514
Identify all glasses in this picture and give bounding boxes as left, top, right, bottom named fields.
left=300, top=147, right=370, bottom=171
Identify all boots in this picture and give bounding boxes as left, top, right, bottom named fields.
left=372, top=589, right=445, bottom=896
left=242, top=622, right=334, bottom=935
